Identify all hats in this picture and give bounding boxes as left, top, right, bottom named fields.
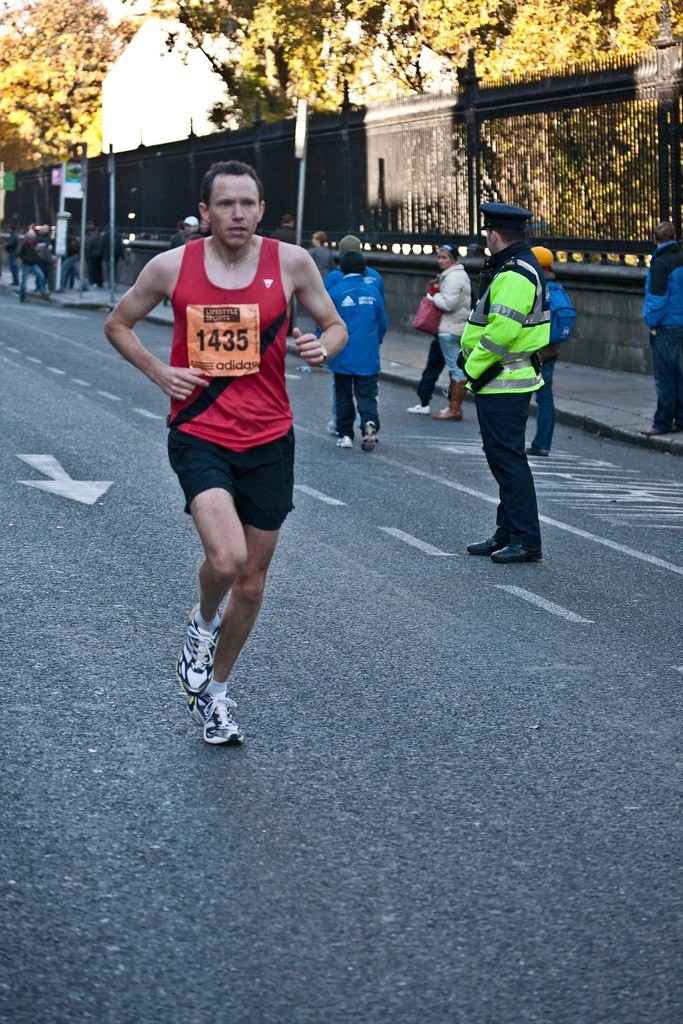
left=531, top=246, right=553, bottom=265
left=478, top=203, right=532, bottom=230
left=339, top=235, right=361, bottom=257
left=340, top=250, right=365, bottom=274
left=440, top=243, right=458, bottom=261
left=184, top=216, right=198, bottom=226
left=282, top=214, right=293, bottom=223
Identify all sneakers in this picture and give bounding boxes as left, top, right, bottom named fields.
left=187, top=690, right=243, bottom=744
left=336, top=436, right=353, bottom=447
left=327, top=420, right=339, bottom=435
left=175, top=603, right=222, bottom=695
left=362, top=420, right=376, bottom=452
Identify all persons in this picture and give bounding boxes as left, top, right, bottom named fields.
left=482, top=246, right=560, bottom=457
left=4, top=223, right=125, bottom=303
left=106, top=160, right=348, bottom=743
left=313, top=235, right=386, bottom=438
left=320, top=250, right=388, bottom=453
left=164, top=216, right=212, bottom=307
left=457, top=202, right=550, bottom=564
left=407, top=242, right=477, bottom=423
left=268, top=214, right=296, bottom=245
left=640, top=221, right=683, bottom=435
left=307, top=231, right=336, bottom=339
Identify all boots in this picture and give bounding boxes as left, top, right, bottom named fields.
left=433, top=379, right=467, bottom=420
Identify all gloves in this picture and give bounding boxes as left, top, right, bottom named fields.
left=428, top=282, right=440, bottom=296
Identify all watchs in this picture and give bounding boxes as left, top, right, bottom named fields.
left=319, top=344, right=328, bottom=364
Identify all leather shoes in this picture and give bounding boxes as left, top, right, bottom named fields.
left=467, top=536, right=503, bottom=554
left=491, top=544, right=542, bottom=563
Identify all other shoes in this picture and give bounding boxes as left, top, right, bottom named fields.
left=407, top=404, right=430, bottom=414
left=526, top=448, right=549, bottom=456
left=671, top=426, right=683, bottom=432
left=641, top=427, right=661, bottom=435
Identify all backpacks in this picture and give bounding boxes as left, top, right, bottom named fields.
left=546, top=281, right=576, bottom=343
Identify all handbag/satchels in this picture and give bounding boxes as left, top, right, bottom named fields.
left=412, top=297, right=442, bottom=334
